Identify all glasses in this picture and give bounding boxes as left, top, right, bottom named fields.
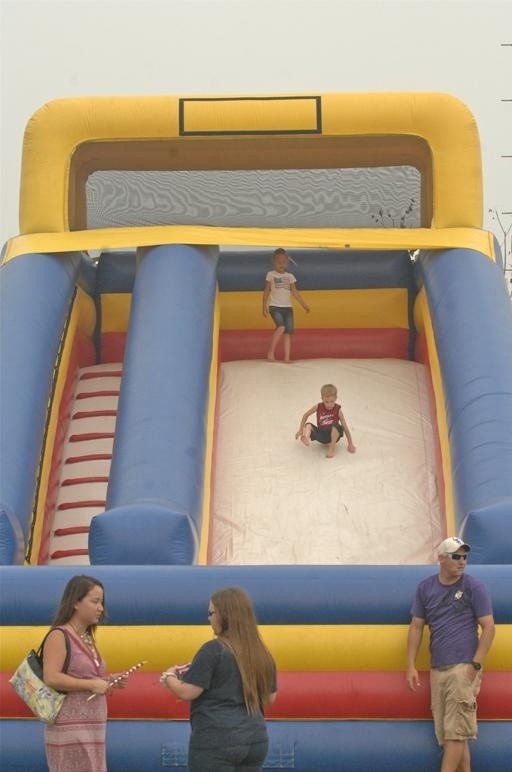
left=447, top=553, right=467, bottom=560
left=208, top=610, right=216, bottom=617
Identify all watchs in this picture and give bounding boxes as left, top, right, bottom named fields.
left=471, top=661, right=481, bottom=670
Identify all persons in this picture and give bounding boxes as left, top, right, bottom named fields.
left=296, top=384, right=355, bottom=458
left=43, top=576, right=129, bottom=772
left=406, top=536, right=495, bottom=772
left=263, top=248, right=310, bottom=363
left=160, top=586, right=277, bottom=772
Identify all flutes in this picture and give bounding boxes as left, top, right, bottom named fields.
left=87, top=660, right=149, bottom=708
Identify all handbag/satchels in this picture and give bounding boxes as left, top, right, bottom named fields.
left=9, top=628, right=71, bottom=726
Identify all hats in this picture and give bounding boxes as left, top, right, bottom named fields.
left=437, top=537, right=472, bottom=558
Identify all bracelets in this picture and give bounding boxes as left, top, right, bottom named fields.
left=160, top=672, right=177, bottom=683
